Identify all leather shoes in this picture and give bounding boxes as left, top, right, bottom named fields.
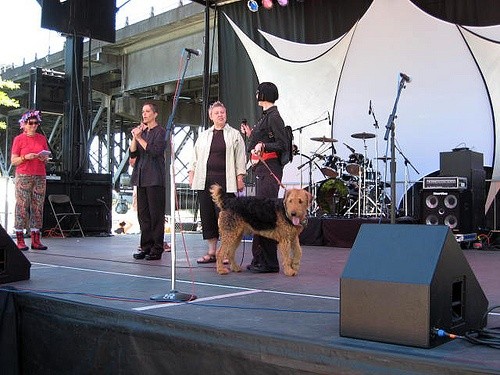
left=133, top=251, right=161, bottom=260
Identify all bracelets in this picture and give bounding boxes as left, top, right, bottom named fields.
left=21, top=155, right=25, bottom=161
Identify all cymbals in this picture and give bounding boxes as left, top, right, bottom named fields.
left=310, top=137, right=339, bottom=143
left=351, top=132, right=375, bottom=139
left=373, top=157, right=397, bottom=161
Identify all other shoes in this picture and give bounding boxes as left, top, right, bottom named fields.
left=247, top=264, right=280, bottom=273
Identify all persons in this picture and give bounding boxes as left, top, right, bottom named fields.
left=129, top=102, right=167, bottom=261
left=241, top=82, right=286, bottom=273
left=187, top=101, right=247, bottom=265
left=10, top=110, right=49, bottom=250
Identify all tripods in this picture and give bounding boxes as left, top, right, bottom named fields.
left=298, top=147, right=391, bottom=219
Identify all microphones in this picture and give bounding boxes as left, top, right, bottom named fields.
left=242, top=118, right=247, bottom=141
left=328, top=112, right=331, bottom=125
left=332, top=144, right=336, bottom=154
left=184, top=48, right=202, bottom=57
left=400, top=73, right=412, bottom=83
left=369, top=100, right=371, bottom=114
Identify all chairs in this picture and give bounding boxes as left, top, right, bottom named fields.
left=49, top=194, right=85, bottom=239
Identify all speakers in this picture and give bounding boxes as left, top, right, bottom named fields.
left=339, top=223, right=489, bottom=349
left=439, top=151, right=486, bottom=234
left=0, top=224, right=32, bottom=284
left=420, top=189, right=473, bottom=248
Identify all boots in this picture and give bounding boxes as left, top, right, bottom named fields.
left=30, top=231, right=48, bottom=249
left=16, top=231, right=29, bottom=251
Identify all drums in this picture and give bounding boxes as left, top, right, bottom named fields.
left=346, top=153, right=365, bottom=175
left=316, top=178, right=362, bottom=217
left=320, top=155, right=342, bottom=176
left=361, top=171, right=381, bottom=186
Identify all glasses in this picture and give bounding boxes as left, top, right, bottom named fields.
left=26, top=121, right=38, bottom=125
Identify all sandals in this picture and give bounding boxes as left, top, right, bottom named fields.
left=197, top=253, right=216, bottom=264
left=223, top=258, right=229, bottom=265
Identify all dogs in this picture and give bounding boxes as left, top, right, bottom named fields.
left=209, top=183, right=312, bottom=276
